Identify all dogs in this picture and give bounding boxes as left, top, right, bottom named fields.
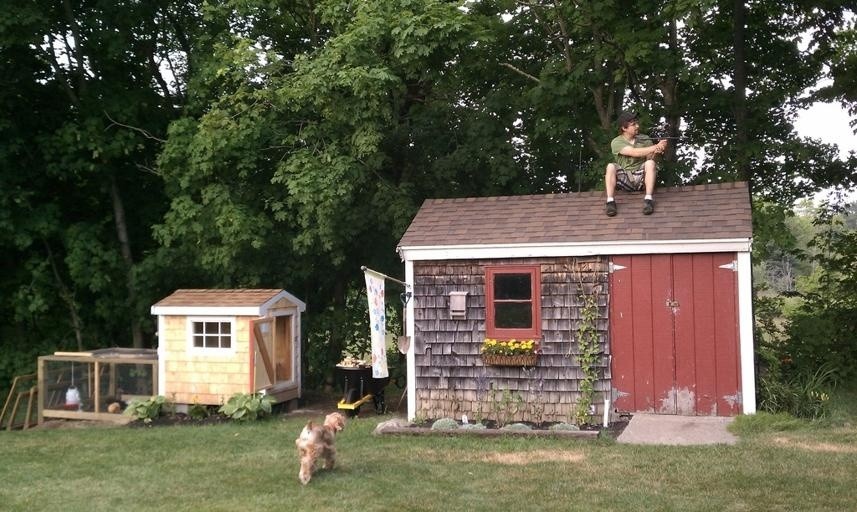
left=295, top=412, right=346, bottom=485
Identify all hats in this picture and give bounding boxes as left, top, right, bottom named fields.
left=617, top=111, right=639, bottom=127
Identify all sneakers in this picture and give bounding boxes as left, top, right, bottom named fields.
left=606, top=199, right=616, bottom=216
left=643, top=199, right=653, bottom=214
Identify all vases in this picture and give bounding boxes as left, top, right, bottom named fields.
left=481, top=353, right=537, bottom=366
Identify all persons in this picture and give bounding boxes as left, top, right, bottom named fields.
left=605, top=111, right=667, bottom=217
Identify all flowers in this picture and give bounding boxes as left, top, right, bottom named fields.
left=480, top=337, right=537, bottom=356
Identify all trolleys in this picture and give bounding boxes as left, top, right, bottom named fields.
left=335, top=365, right=395, bottom=417
